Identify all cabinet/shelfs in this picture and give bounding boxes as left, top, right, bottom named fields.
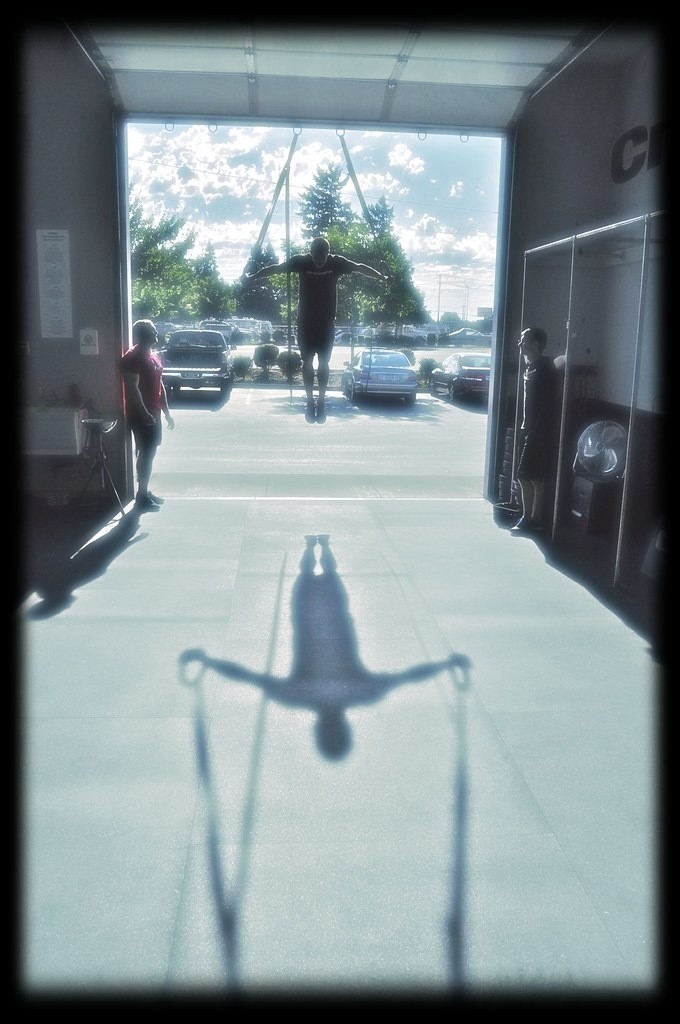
left=562, top=475, right=619, bottom=556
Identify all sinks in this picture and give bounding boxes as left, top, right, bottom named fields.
left=23, top=404, right=88, bottom=457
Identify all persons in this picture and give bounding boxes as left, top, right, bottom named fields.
left=510, top=321, right=585, bottom=535
left=120, top=319, right=174, bottom=513
left=240, top=237, right=395, bottom=424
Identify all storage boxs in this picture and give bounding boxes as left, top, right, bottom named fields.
left=23, top=408, right=89, bottom=450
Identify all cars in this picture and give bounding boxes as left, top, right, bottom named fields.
left=341, top=349, right=418, bottom=405
left=427, top=352, right=492, bottom=401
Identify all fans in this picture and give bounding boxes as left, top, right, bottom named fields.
left=573, top=420, right=630, bottom=479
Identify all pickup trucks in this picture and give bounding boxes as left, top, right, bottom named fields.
left=154, top=329, right=234, bottom=400
